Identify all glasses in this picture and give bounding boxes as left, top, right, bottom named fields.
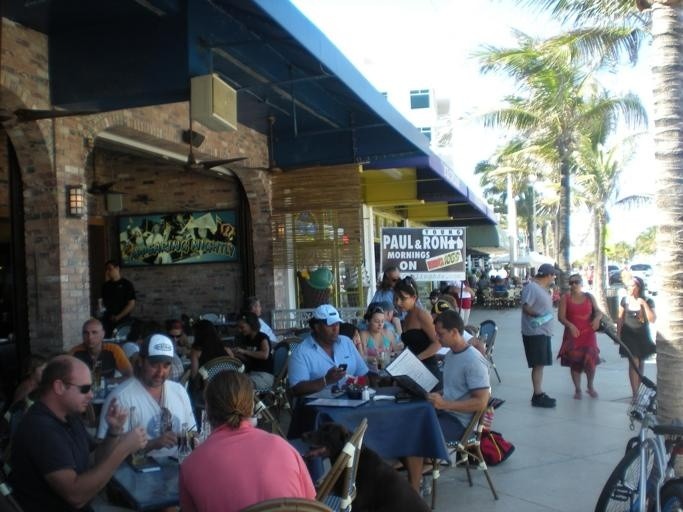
left=567, top=280, right=582, bottom=285
left=61, top=382, right=96, bottom=394
left=168, top=334, right=183, bottom=338
left=371, top=308, right=385, bottom=314
left=162, top=406, right=173, bottom=434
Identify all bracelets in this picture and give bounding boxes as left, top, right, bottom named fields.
left=105, top=429, right=119, bottom=438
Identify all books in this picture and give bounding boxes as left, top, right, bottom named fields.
left=385, top=345, right=440, bottom=397
left=304, top=397, right=369, bottom=408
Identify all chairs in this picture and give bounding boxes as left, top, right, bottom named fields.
left=1, top=473, right=27, bottom=512
left=293, top=328, right=315, bottom=340
left=193, top=357, right=245, bottom=409
left=417, top=395, right=499, bottom=511
left=252, top=340, right=293, bottom=424
left=476, top=321, right=501, bottom=385
left=280, top=337, right=298, bottom=345
left=235, top=498, right=332, bottom=512
left=313, top=416, right=368, bottom=511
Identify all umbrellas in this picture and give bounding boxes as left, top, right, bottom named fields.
left=514, top=251, right=552, bottom=264
left=488, top=252, right=510, bottom=262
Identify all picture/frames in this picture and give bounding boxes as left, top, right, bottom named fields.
left=116, top=207, right=242, bottom=269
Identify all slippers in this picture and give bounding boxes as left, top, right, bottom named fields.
left=586, top=389, right=598, bottom=399
left=573, top=391, right=584, bottom=400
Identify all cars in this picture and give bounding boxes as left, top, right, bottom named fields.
left=609, top=264, right=621, bottom=285
left=629, top=264, right=653, bottom=290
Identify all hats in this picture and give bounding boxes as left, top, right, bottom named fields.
left=139, top=334, right=175, bottom=364
left=534, top=264, right=554, bottom=278
left=435, top=301, right=449, bottom=314
left=305, top=267, right=334, bottom=290
left=311, top=304, right=346, bottom=326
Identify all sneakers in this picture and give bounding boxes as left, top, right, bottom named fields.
left=418, top=475, right=433, bottom=499
left=531, top=392, right=557, bottom=408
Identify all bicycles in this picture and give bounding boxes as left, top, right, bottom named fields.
left=593, top=316, right=682, bottom=512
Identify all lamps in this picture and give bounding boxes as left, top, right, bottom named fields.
left=64, top=187, right=86, bottom=218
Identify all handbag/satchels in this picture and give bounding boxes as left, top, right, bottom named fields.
left=587, top=292, right=607, bottom=331
left=457, top=431, right=514, bottom=465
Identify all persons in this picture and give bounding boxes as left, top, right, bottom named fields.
left=380, top=300, right=403, bottom=344
left=15, top=354, right=148, bottom=512
left=369, top=266, right=424, bottom=321
left=470, top=259, right=530, bottom=304
left=113, top=328, right=144, bottom=379
left=245, top=296, right=276, bottom=341
left=178, top=369, right=317, bottom=512
left=359, top=302, right=404, bottom=364
left=166, top=319, right=192, bottom=357
left=339, top=323, right=364, bottom=355
left=617, top=275, right=656, bottom=405
left=69, top=319, right=132, bottom=385
left=449, top=280, right=475, bottom=326
left=403, top=309, right=491, bottom=502
left=430, top=330, right=484, bottom=393
left=343, top=243, right=371, bottom=307
left=186, top=319, right=234, bottom=432
left=520, top=263, right=561, bottom=408
left=231, top=312, right=271, bottom=371
left=13, top=353, right=47, bottom=401
left=146, top=223, right=171, bottom=265
left=556, top=273, right=602, bottom=399
left=96, top=332, right=200, bottom=456
left=98, top=260, right=135, bottom=337
left=394, top=276, right=442, bottom=390
left=430, top=291, right=449, bottom=320
left=288, top=303, right=371, bottom=435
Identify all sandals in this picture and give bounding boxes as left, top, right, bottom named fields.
left=631, top=392, right=639, bottom=405
left=366, top=453, right=396, bottom=474
